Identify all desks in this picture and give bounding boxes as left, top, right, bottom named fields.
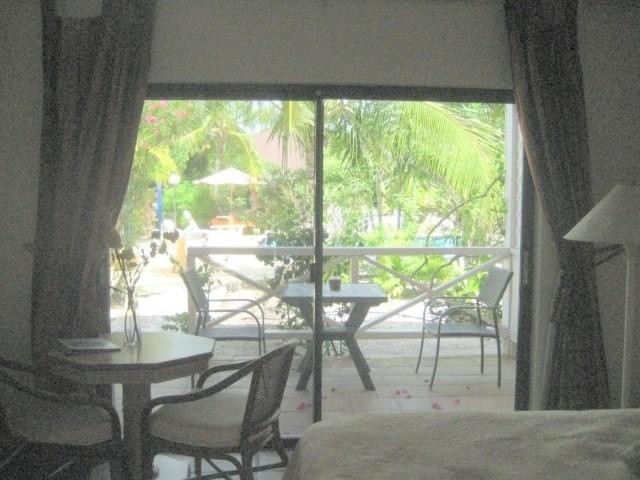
left=282, top=282, right=388, bottom=390
left=50, top=331, right=217, bottom=478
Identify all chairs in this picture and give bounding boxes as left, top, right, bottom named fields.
left=1, top=358, right=126, bottom=480
left=415, top=267, right=514, bottom=390
left=141, top=339, right=298, bottom=479
left=180, top=266, right=269, bottom=392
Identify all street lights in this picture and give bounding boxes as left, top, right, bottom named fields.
left=168, top=174, right=181, bottom=229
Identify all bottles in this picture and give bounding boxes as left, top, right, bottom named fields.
left=124, top=301, right=143, bottom=348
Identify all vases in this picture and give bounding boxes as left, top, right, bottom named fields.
left=124, top=297, right=140, bottom=346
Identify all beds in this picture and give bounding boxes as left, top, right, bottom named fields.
left=282, top=406, right=638, bottom=478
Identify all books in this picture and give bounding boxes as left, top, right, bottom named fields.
left=55, top=338, right=121, bottom=355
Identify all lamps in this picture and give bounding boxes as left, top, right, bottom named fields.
left=563, top=184, right=640, bottom=407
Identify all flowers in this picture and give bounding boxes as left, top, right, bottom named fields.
left=102, top=230, right=180, bottom=343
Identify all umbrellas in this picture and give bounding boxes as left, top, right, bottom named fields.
left=191, top=165, right=269, bottom=217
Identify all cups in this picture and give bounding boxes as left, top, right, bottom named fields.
left=329, top=279, right=341, bottom=291
